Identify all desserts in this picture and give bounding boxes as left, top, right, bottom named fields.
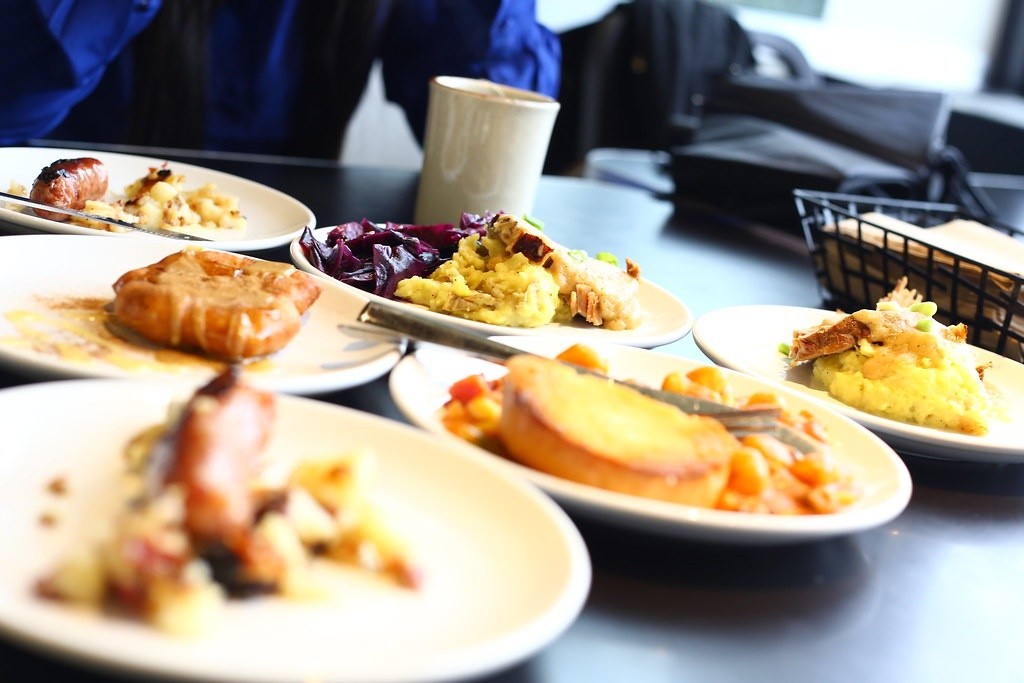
left=496, top=353, right=738, bottom=512
left=110, top=244, right=321, bottom=360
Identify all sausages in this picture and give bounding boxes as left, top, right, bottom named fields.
left=27, top=157, right=110, bottom=220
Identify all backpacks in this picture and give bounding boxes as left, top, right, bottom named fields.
left=582, top=0, right=762, bottom=155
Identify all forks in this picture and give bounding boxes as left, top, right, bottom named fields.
left=339, top=323, right=781, bottom=433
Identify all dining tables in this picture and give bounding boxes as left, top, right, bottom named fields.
left=0, top=147, right=1024, bottom=683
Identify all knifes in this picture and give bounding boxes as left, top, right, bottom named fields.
left=0, top=191, right=213, bottom=242
left=358, top=300, right=814, bottom=456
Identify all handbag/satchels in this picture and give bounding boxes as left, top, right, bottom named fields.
left=652, top=83, right=1008, bottom=248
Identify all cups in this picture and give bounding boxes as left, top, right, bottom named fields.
left=414, top=75, right=561, bottom=229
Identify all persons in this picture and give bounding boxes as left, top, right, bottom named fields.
left=0, top=0, right=559, bottom=161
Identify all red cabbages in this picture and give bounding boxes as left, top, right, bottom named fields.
left=296, top=211, right=506, bottom=298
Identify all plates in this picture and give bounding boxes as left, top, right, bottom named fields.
left=388, top=336, right=914, bottom=535
left=0, top=379, right=594, bottom=682
left=0, top=147, right=317, bottom=252
left=696, top=305, right=1024, bottom=467
left=290, top=222, right=694, bottom=349
left=0, top=234, right=407, bottom=393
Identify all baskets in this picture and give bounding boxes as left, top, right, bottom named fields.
left=791, top=186, right=1024, bottom=367
